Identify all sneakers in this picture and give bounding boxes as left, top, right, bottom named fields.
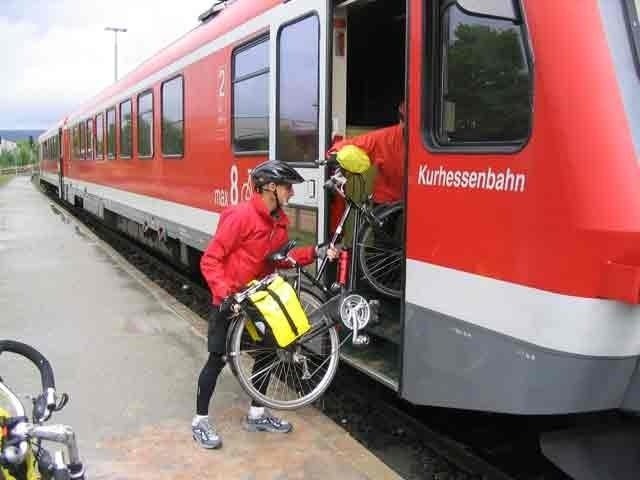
left=243, top=411, right=295, bottom=434
left=191, top=416, right=223, bottom=449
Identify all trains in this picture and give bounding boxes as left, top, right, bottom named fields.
left=25, top=2, right=637, bottom=423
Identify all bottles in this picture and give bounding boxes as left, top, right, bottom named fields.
left=336, top=246, right=351, bottom=287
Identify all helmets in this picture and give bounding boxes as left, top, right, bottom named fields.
left=250, top=159, right=305, bottom=189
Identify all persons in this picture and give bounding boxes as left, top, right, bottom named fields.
left=190, top=160, right=342, bottom=449
left=325, top=102, right=406, bottom=326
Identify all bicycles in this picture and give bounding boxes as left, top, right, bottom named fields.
left=217, top=145, right=405, bottom=412
